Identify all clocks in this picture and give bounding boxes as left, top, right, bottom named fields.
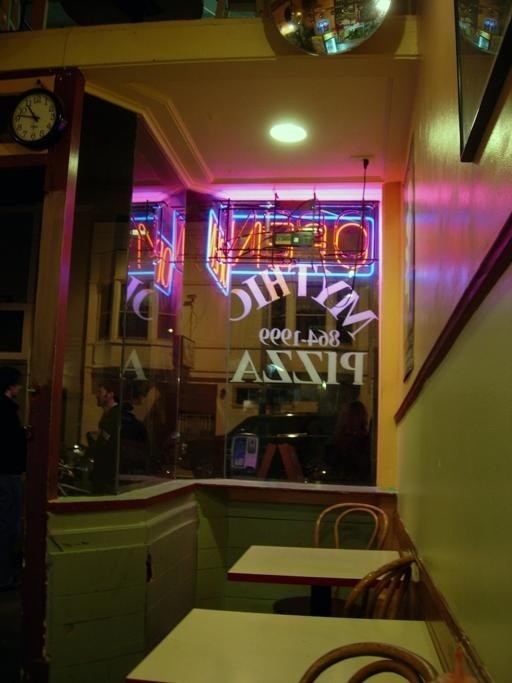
left=6, top=84, right=65, bottom=152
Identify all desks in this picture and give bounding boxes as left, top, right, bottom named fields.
left=121, top=603, right=483, bottom=683
left=225, top=540, right=428, bottom=619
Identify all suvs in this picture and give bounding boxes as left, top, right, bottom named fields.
left=180, top=411, right=340, bottom=484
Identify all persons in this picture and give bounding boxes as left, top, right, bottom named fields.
left=1, top=365, right=26, bottom=496
left=318, top=376, right=368, bottom=485
left=91, top=379, right=148, bottom=492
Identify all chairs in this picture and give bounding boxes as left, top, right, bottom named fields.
left=336, top=555, right=422, bottom=620
left=269, top=500, right=393, bottom=616
left=293, top=637, right=448, bottom=683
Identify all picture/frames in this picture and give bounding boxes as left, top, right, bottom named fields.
left=448, top=0, right=512, bottom=162
